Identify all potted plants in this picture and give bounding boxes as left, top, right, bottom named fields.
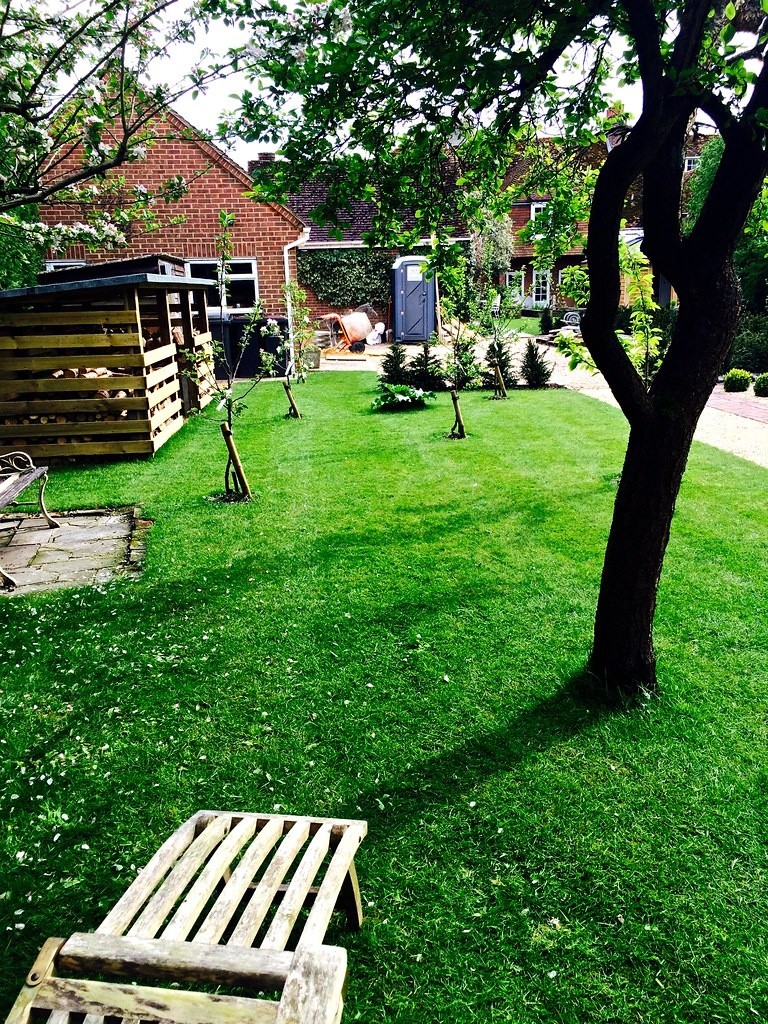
left=303, top=343, right=321, bottom=369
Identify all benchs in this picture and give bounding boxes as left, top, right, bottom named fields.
left=0, top=450, right=61, bottom=589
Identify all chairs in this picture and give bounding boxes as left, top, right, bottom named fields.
left=489, top=294, right=499, bottom=319
left=0, top=808, right=368, bottom=1024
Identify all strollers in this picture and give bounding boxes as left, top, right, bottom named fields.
left=316, top=303, right=381, bottom=355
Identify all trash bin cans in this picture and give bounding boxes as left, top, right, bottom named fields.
left=207, top=311, right=289, bottom=379
